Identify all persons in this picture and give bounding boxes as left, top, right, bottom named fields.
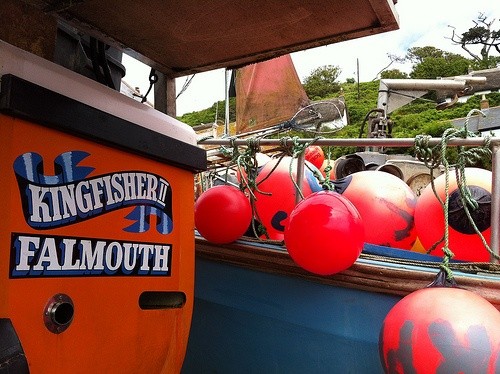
left=338, top=88, right=345, bottom=99
